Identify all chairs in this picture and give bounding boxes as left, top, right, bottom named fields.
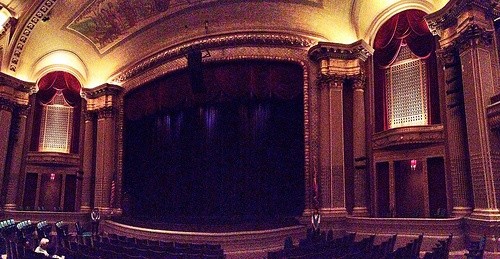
left=0, top=219, right=454, bottom=259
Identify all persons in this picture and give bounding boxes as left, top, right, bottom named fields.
left=311, top=208, right=321, bottom=235
left=90, top=208, right=101, bottom=236
left=51, top=246, right=66, bottom=259
left=33, top=237, right=50, bottom=258
